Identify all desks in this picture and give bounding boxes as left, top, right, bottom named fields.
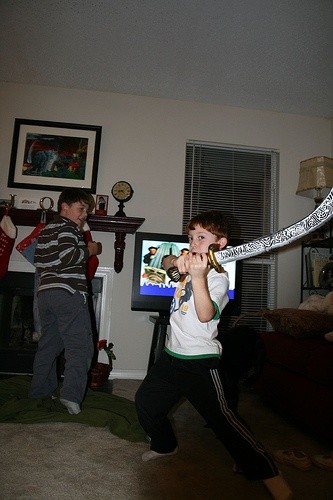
left=147, top=316, right=240, bottom=405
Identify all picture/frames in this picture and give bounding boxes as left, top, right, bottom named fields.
left=6, top=118, right=102, bottom=194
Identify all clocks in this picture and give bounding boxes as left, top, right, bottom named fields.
left=40, top=196, right=54, bottom=210
left=110, top=180, right=133, bottom=218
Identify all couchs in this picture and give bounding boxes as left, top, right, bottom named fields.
left=250, top=329, right=333, bottom=449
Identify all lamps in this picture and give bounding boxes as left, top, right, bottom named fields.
left=296, top=156, right=333, bottom=210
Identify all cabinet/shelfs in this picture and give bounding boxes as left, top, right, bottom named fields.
left=300, top=225, right=333, bottom=302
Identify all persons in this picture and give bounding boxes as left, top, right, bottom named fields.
left=30, top=187, right=98, bottom=414
left=99, top=198, right=106, bottom=211
left=136, top=211, right=293, bottom=500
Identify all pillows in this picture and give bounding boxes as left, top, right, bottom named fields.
left=262, top=307, right=333, bottom=342
